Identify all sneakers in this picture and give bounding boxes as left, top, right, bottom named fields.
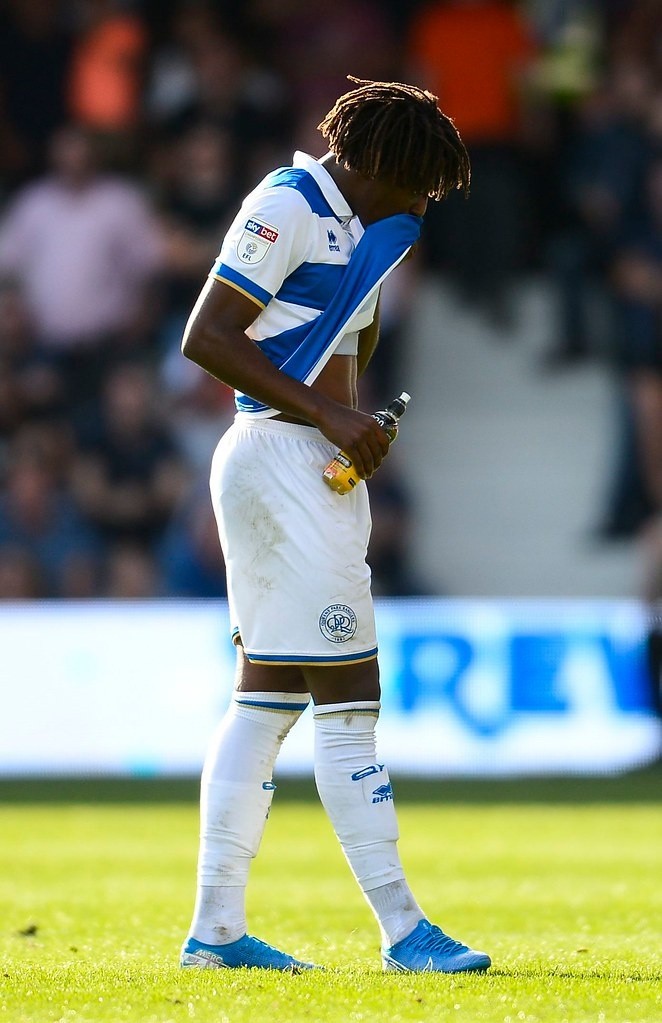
left=181, top=933, right=327, bottom=977
left=380, top=919, right=492, bottom=974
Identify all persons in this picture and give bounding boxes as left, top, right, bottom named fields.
left=0, top=0, right=662, bottom=600
left=178, top=76, right=492, bottom=974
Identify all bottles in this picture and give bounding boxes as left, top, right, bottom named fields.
left=323, top=391, right=411, bottom=495
